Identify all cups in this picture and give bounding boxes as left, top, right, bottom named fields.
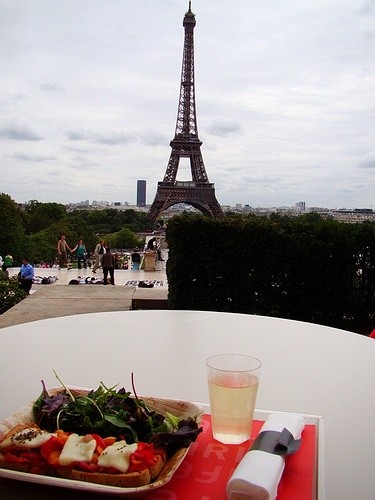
left=206, top=353, right=261, bottom=446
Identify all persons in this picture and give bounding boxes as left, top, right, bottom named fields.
left=112, top=248, right=128, bottom=270
left=71, top=239, right=87, bottom=269
left=5, top=253, right=12, bottom=259
left=36, top=260, right=59, bottom=268
left=132, top=249, right=141, bottom=269
left=20, top=258, right=34, bottom=295
left=148, top=238, right=165, bottom=261
left=92, top=240, right=106, bottom=273
left=58, top=235, right=71, bottom=271
left=101, top=247, right=115, bottom=285
left=2, top=265, right=8, bottom=277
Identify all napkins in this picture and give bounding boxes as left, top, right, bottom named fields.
left=226, top=413, right=305, bottom=500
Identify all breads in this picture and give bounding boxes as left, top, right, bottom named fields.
left=0, top=425, right=167, bottom=486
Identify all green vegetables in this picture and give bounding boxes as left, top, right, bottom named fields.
left=29, top=370, right=204, bottom=458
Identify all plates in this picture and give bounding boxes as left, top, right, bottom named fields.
left=0, top=387, right=204, bottom=494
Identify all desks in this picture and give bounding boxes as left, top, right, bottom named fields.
left=0, top=310, right=375, bottom=500
